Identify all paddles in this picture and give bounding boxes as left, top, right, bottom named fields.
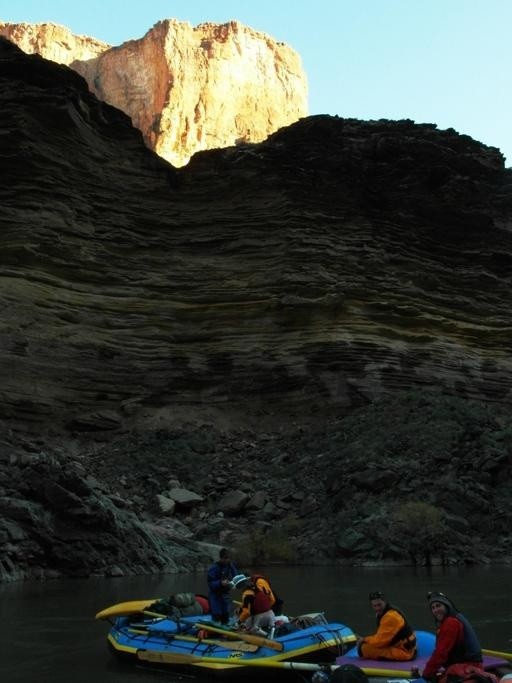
left=128, top=629, right=258, bottom=653
left=144, top=611, right=284, bottom=652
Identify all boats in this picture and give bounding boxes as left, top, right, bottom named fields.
left=344, top=622, right=512, bottom=682
left=95, top=592, right=357, bottom=676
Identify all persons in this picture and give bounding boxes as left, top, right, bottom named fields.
left=205, top=546, right=239, bottom=626
left=230, top=575, right=285, bottom=641
left=354, top=589, right=416, bottom=662
left=421, top=591, right=484, bottom=683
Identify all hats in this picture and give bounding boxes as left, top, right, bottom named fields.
left=427, top=592, right=452, bottom=607
left=232, top=573, right=248, bottom=587
